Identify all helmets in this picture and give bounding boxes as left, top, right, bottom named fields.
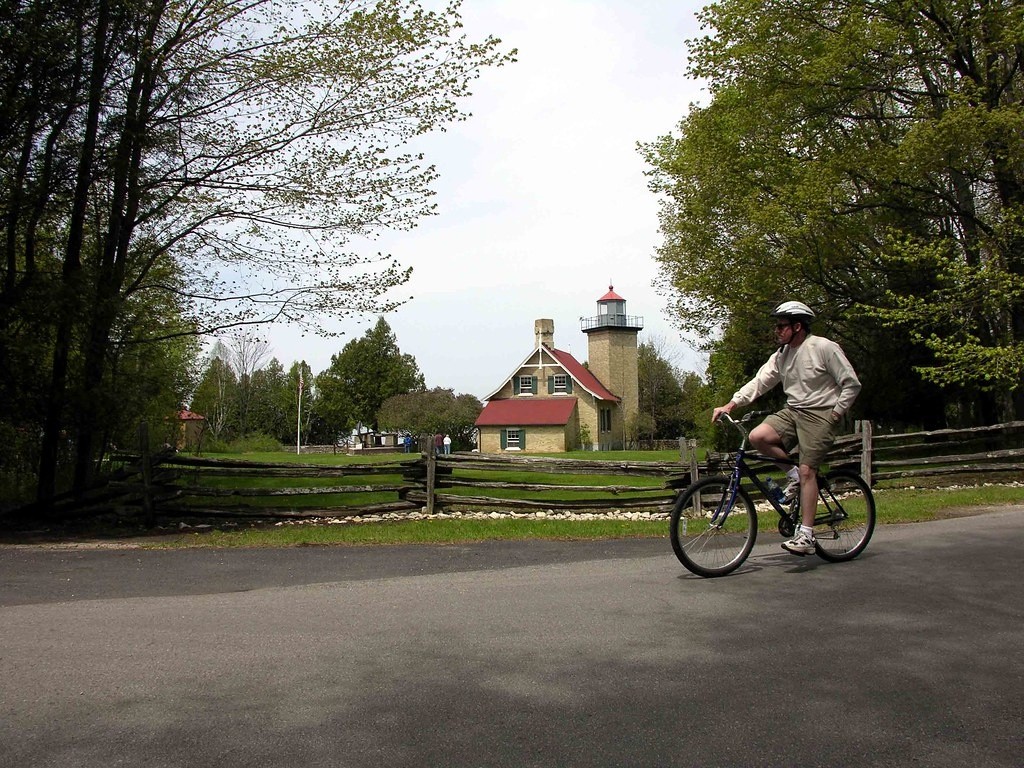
left=770, top=301, right=815, bottom=320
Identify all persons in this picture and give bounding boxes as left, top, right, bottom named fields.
left=405, top=434, right=411, bottom=453
left=435, top=431, right=443, bottom=454
left=712, top=301, right=861, bottom=556
left=443, top=434, right=451, bottom=454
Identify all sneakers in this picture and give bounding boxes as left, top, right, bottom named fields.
left=781, top=530, right=816, bottom=556
left=781, top=478, right=801, bottom=503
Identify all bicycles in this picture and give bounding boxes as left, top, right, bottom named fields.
left=669, top=410, right=877, bottom=577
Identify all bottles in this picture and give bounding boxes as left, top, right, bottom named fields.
left=766, top=477, right=788, bottom=504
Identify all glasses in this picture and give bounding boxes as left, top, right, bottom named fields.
left=774, top=323, right=792, bottom=329
